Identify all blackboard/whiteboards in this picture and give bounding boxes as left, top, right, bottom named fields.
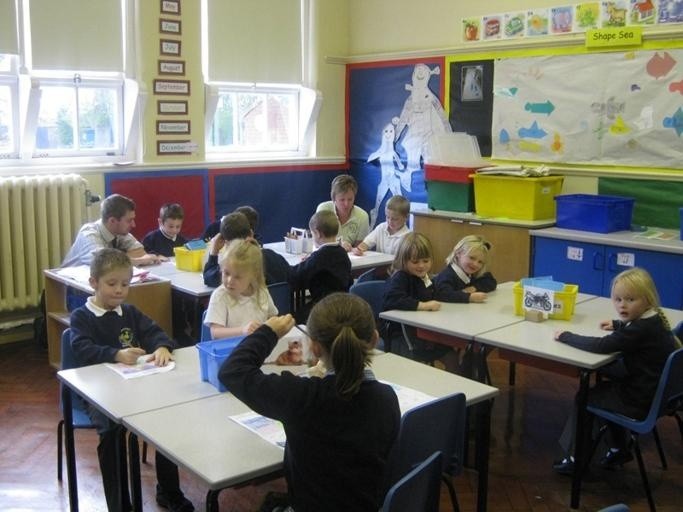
left=439, top=40, right=683, bottom=176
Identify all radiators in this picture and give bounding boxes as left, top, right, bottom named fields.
left=0, top=171, right=93, bottom=314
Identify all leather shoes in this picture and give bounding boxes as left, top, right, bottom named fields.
left=586, top=446, right=636, bottom=477
left=551, top=455, right=582, bottom=473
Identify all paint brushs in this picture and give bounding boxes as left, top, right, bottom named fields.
left=346, top=235, right=365, bottom=257
left=120, top=336, right=134, bottom=348
left=294, top=321, right=317, bottom=341
left=286, top=230, right=299, bottom=240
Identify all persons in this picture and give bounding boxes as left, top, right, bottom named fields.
left=70, top=249, right=194, bottom=512
left=381, top=232, right=496, bottom=369
left=142, top=203, right=189, bottom=256
left=317, top=175, right=368, bottom=251
left=288, top=210, right=352, bottom=318
left=61, top=194, right=162, bottom=267
left=354, top=195, right=413, bottom=282
left=202, top=205, right=292, bottom=287
left=551, top=265, right=682, bottom=473
left=217, top=291, right=401, bottom=512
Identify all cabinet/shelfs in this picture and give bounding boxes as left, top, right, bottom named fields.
left=42, top=263, right=172, bottom=373
left=528, top=236, right=683, bottom=310
left=410, top=213, right=556, bottom=285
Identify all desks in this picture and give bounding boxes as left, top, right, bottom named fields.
left=132, top=235, right=395, bottom=346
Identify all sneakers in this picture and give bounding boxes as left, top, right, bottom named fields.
left=155, top=488, right=195, bottom=511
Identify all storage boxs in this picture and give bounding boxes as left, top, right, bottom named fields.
left=468, top=173, right=564, bottom=222
left=426, top=180, right=475, bottom=213
left=173, top=247, right=206, bottom=273
left=553, top=193, right=636, bottom=235
